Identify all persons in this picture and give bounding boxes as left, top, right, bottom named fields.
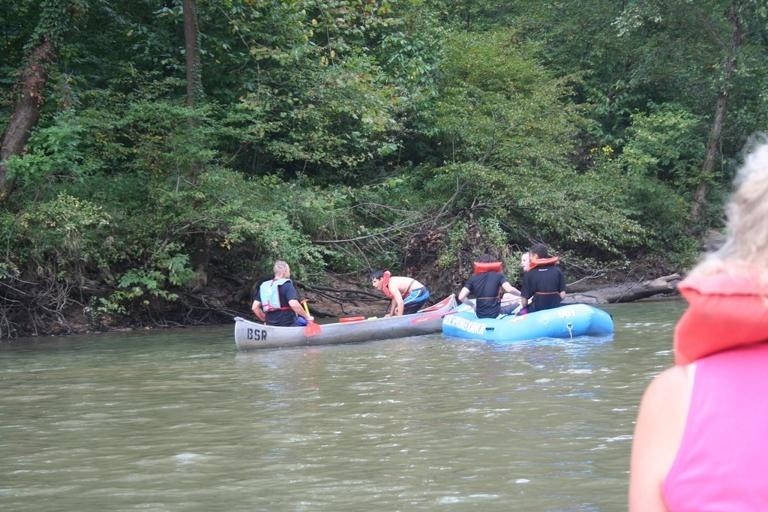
left=458, top=254, right=522, bottom=319
left=520, top=252, right=530, bottom=272
left=252, top=260, right=314, bottom=326
left=370, top=270, right=429, bottom=317
left=517, top=244, right=566, bottom=315
left=628, top=131, right=768, bottom=512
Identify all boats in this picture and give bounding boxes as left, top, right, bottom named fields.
left=231, top=292, right=458, bottom=351
left=443, top=298, right=616, bottom=345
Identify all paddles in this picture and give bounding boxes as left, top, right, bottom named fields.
left=411, top=298, right=523, bottom=324
left=300, top=298, right=321, bottom=337
